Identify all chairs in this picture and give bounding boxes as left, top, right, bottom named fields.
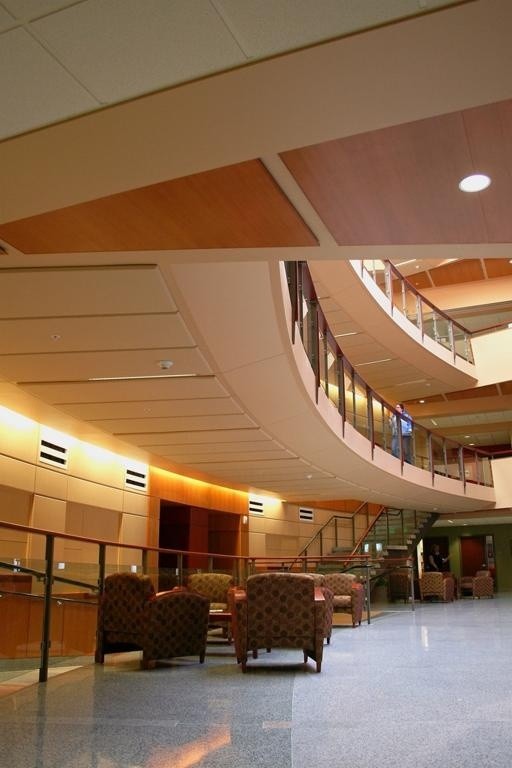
left=387, top=567, right=495, bottom=605
left=93, top=569, right=365, bottom=674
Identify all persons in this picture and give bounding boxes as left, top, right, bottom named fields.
left=428, top=544, right=447, bottom=572
left=389, top=404, right=413, bottom=464
